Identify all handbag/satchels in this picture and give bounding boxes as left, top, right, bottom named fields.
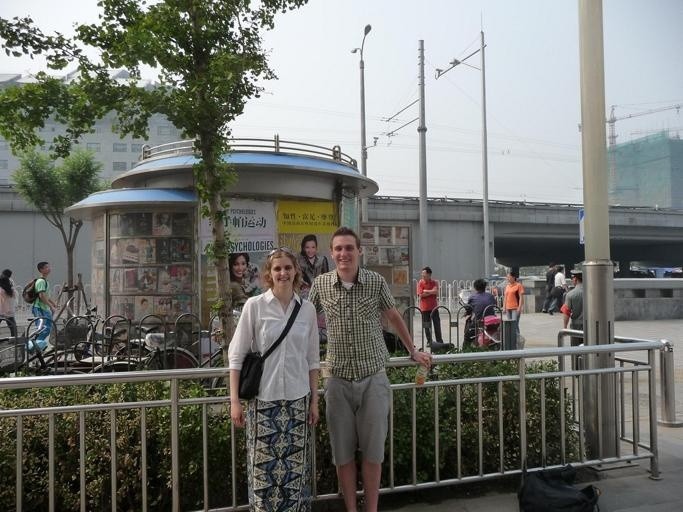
left=238, top=351, right=264, bottom=400
left=516, top=463, right=601, bottom=512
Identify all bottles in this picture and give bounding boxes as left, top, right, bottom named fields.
left=415, top=366, right=427, bottom=384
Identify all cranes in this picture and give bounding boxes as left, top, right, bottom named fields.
left=578, top=104, right=683, bottom=205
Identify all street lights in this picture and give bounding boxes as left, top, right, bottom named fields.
left=350, top=24, right=379, bottom=222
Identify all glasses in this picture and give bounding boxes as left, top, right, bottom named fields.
left=266, top=248, right=298, bottom=256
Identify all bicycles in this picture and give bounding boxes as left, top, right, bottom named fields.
left=0, top=297, right=225, bottom=388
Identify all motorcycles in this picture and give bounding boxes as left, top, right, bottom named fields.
left=459, top=294, right=501, bottom=349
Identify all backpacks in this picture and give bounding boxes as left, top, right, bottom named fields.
left=22, top=278, right=48, bottom=304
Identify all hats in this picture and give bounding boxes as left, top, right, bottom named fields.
left=570, top=270, right=582, bottom=277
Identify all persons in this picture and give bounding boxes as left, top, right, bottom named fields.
left=307, top=225, right=432, bottom=512
left=154, top=211, right=171, bottom=230
left=296, top=233, right=329, bottom=291
left=31, top=262, right=58, bottom=340
left=227, top=253, right=265, bottom=312
left=461, top=271, right=525, bottom=351
left=0, top=274, right=18, bottom=337
left=3, top=269, right=15, bottom=286
left=542, top=261, right=584, bottom=377
left=417, top=266, right=444, bottom=346
left=229, top=248, right=323, bottom=512
left=108, top=238, right=193, bottom=320
